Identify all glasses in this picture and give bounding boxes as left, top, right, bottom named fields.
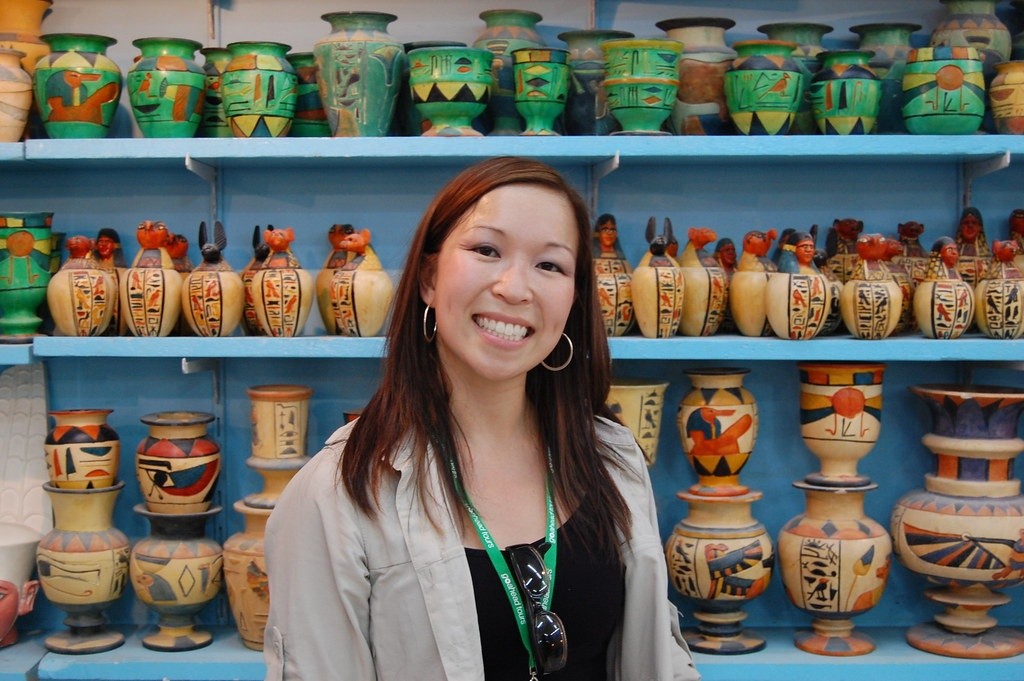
left=506, top=543, right=567, bottom=675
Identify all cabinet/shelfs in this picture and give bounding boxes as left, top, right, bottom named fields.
left=0, top=135, right=1023, bottom=680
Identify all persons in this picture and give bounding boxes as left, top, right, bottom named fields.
left=592, top=214, right=625, bottom=259
left=263, top=152, right=701, bottom=681
left=0, top=578, right=39, bottom=647
left=709, top=209, right=1024, bottom=280
left=90, top=227, right=124, bottom=270
